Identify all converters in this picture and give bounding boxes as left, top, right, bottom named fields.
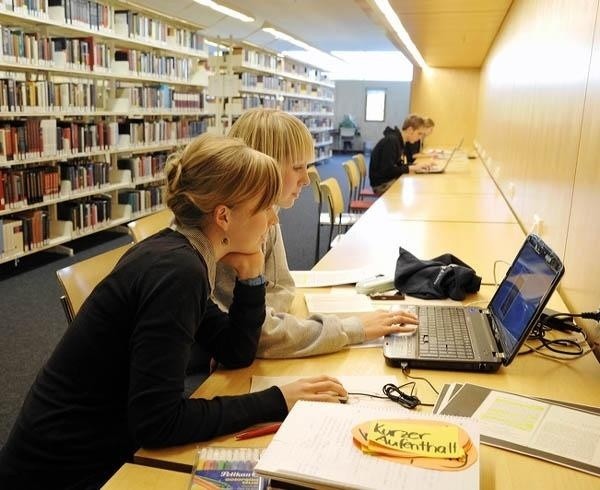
left=540, top=308, right=573, bottom=331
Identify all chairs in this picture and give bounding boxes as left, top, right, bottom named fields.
left=56, top=242, right=135, bottom=325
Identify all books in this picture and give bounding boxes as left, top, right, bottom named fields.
left=1, top=1, right=335, bottom=267
left=254, top=398, right=482, bottom=490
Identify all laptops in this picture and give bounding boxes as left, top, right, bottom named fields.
left=383, top=234, right=565, bottom=372
left=433, top=137, right=464, bottom=160
left=415, top=144, right=457, bottom=173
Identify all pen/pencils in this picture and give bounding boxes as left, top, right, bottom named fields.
left=197, top=447, right=265, bottom=471
left=234, top=423, right=281, bottom=441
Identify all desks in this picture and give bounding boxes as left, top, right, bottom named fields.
left=100, top=151, right=600, bottom=490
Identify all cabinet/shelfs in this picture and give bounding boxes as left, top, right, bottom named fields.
left=0, top=0, right=209, bottom=264
left=206, top=34, right=336, bottom=167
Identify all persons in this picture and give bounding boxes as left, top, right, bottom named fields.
left=369, top=114, right=437, bottom=198
left=210, top=106, right=420, bottom=361
left=402, top=118, right=440, bottom=164
left=1, top=130, right=351, bottom=490
